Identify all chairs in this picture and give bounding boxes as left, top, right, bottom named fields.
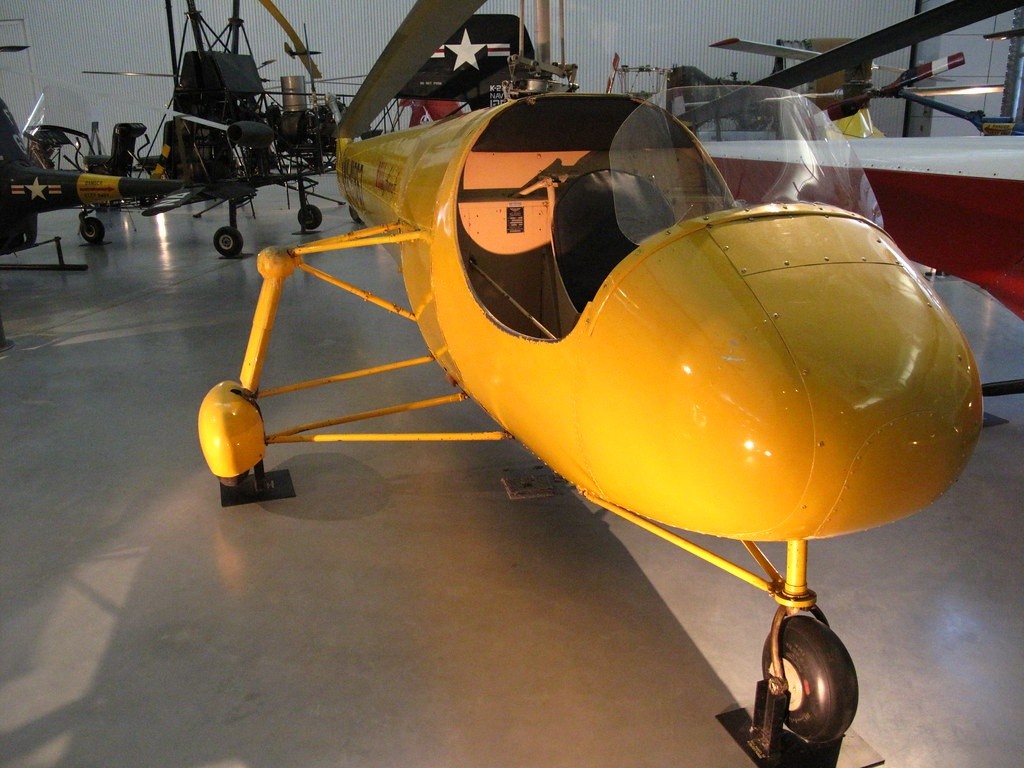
left=552, top=171, right=679, bottom=325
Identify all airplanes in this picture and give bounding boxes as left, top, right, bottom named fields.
left=0, top=1, right=1024, bottom=401
left=196, top=0, right=985, bottom=747
